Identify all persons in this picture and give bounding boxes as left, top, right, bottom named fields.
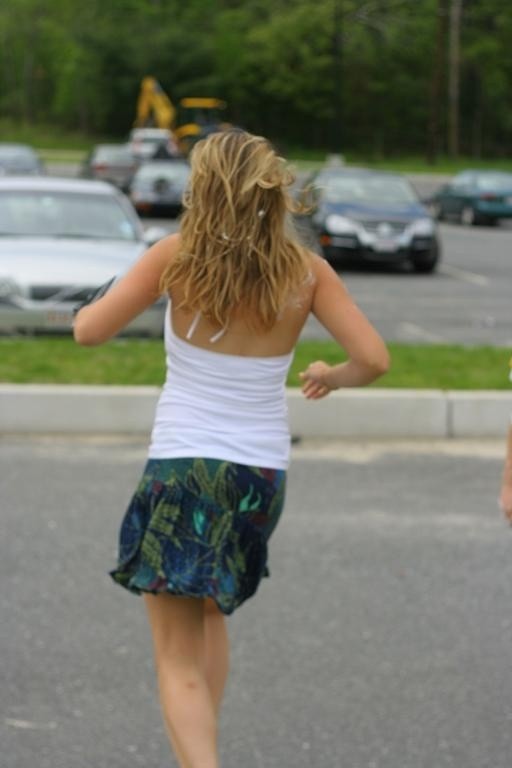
left=72, top=126, right=390, bottom=767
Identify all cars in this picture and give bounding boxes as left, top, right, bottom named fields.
left=425, top=171, right=512, bottom=226
